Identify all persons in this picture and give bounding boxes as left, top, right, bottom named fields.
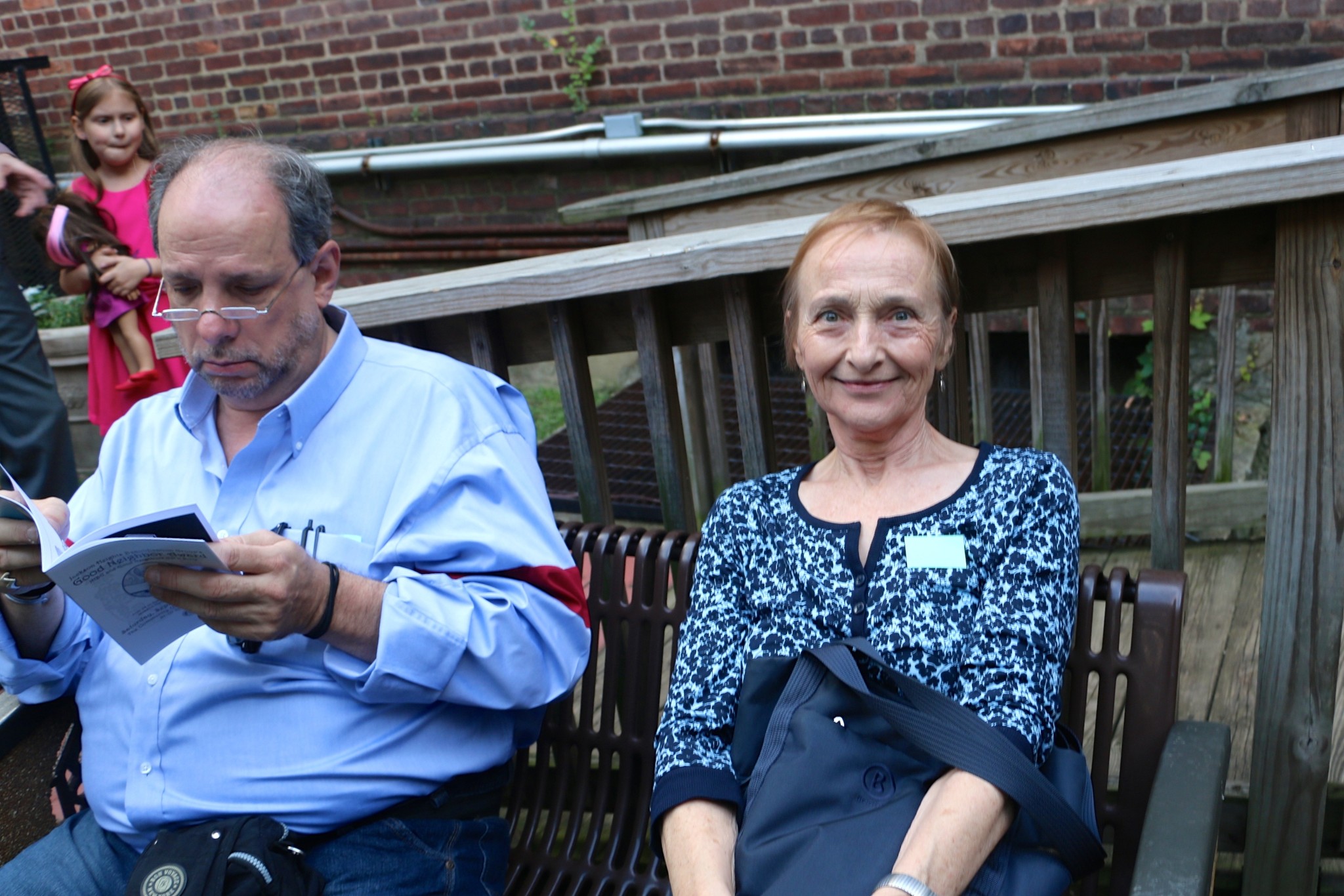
left=0, top=140, right=593, bottom=896
left=644, top=188, right=1101, bottom=896
left=0, top=102, right=82, bottom=511
left=59, top=64, right=191, bottom=440
left=28, top=178, right=161, bottom=393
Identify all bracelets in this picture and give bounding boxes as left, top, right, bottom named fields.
left=3, top=585, right=57, bottom=605
left=142, top=257, right=153, bottom=278
left=872, top=874, right=933, bottom=896
left=303, top=561, right=338, bottom=640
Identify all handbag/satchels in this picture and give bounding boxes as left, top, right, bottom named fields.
left=708, top=636, right=1106, bottom=896
left=126, top=814, right=324, bottom=896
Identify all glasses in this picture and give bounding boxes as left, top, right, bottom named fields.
left=151, top=262, right=305, bottom=322
left=225, top=521, right=290, bottom=655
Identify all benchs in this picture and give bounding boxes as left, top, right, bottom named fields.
left=1, top=522, right=1231, bottom=895
